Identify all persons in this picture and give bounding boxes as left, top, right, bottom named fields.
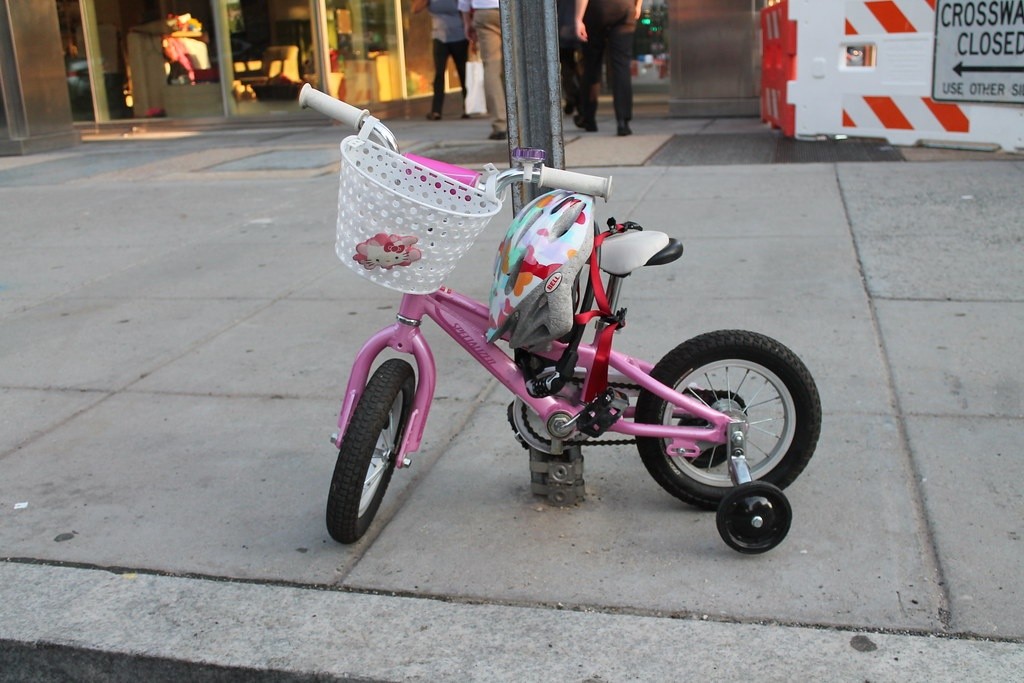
left=558, top=0, right=643, bottom=136
left=426, top=0, right=508, bottom=140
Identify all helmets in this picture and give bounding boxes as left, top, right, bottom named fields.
left=486, top=188, right=594, bottom=340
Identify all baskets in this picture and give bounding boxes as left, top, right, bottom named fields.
left=331, top=135, right=501, bottom=294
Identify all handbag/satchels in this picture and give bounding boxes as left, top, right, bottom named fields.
left=463, top=41, right=489, bottom=114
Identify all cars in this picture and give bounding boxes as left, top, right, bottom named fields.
left=64, top=0, right=388, bottom=112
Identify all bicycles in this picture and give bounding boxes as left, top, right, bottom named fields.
left=298, top=83, right=823, bottom=555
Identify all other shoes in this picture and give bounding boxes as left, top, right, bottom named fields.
left=425, top=111, right=441, bottom=120
left=489, top=131, right=505, bottom=139
left=616, top=120, right=632, bottom=135
left=573, top=114, right=596, bottom=132
left=461, top=113, right=470, bottom=119
left=563, top=102, right=577, bottom=114
